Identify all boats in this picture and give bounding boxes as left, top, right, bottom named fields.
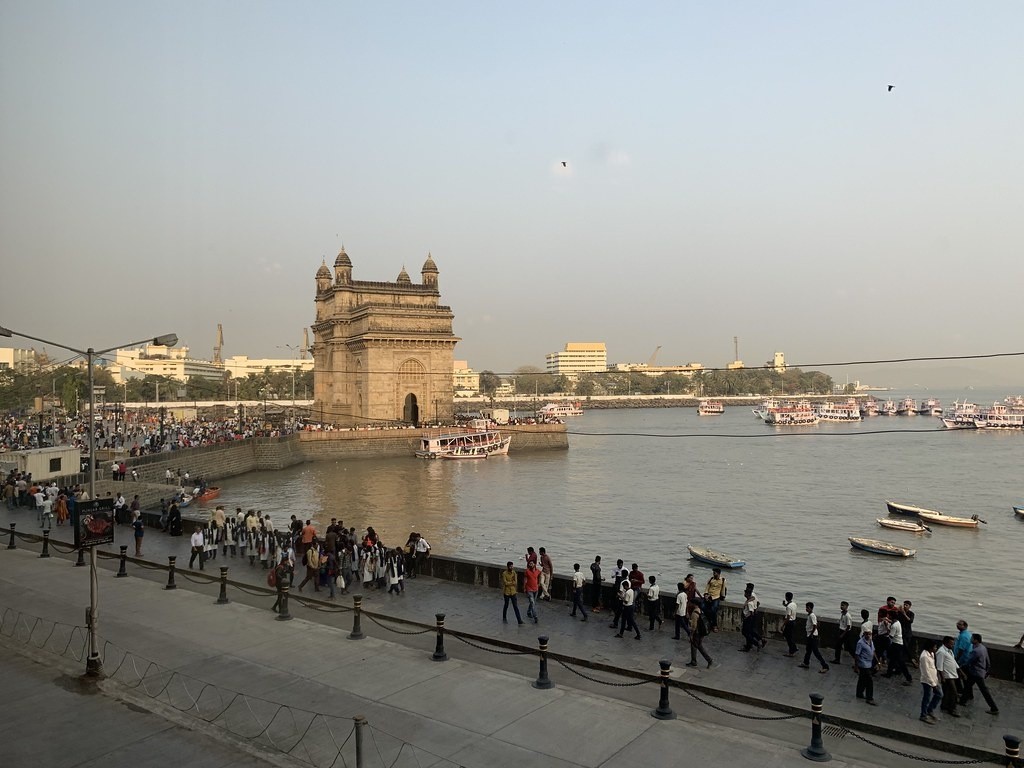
left=685, top=544, right=745, bottom=569
left=178, top=495, right=194, bottom=507
left=898, top=396, right=918, bottom=418
left=848, top=536, right=918, bottom=558
left=696, top=401, right=724, bottom=417
left=414, top=430, right=512, bottom=461
left=752, top=396, right=898, bottom=434
left=921, top=397, right=943, bottom=417
left=940, top=395, right=1024, bottom=430
left=535, top=403, right=584, bottom=417
left=198, top=486, right=222, bottom=501
left=876, top=517, right=933, bottom=533
left=884, top=499, right=943, bottom=517
left=917, top=512, right=988, bottom=528
left=1012, top=506, right=1024, bottom=515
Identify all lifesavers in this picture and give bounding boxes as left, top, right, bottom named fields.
left=807, top=419, right=814, bottom=423
left=986, top=423, right=991, bottom=426
left=834, top=416, right=838, bottom=419
left=818, top=414, right=822, bottom=418
left=794, top=420, right=798, bottom=424
left=772, top=420, right=776, bottom=424
left=500, top=443, right=504, bottom=448
left=824, top=415, right=827, bottom=418
left=1001, top=424, right=1006, bottom=427
left=961, top=422, right=965, bottom=425
left=955, top=422, right=958, bottom=425
left=994, top=424, right=998, bottom=427
left=479, top=448, right=485, bottom=453
left=778, top=420, right=782, bottom=424
left=839, top=416, right=858, bottom=420
left=799, top=419, right=806, bottom=423
left=967, top=422, right=971, bottom=426
left=493, top=444, right=498, bottom=450
left=488, top=446, right=492, bottom=452
left=1009, top=424, right=1024, bottom=428
left=424, top=454, right=429, bottom=459
left=788, top=421, right=792, bottom=424
left=430, top=453, right=436, bottom=459
left=783, top=420, right=786, bottom=424
left=829, top=416, right=833, bottom=419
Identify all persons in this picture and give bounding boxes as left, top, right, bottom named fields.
left=270, top=557, right=295, bottom=614
left=831, top=602, right=855, bottom=665
left=501, top=562, right=525, bottom=624
left=615, top=581, right=643, bottom=641
left=918, top=643, right=941, bottom=724
left=854, top=633, right=881, bottom=707
left=686, top=599, right=722, bottom=671
left=643, top=576, right=664, bottom=632
left=132, top=515, right=144, bottom=558
left=188, top=526, right=205, bottom=569
left=799, top=603, right=830, bottom=674
left=952, top=621, right=973, bottom=666
left=537, top=547, right=553, bottom=602
left=0, top=408, right=565, bottom=626
left=568, top=556, right=1000, bottom=726
left=670, top=583, right=691, bottom=640
left=936, top=635, right=968, bottom=717
left=301, top=520, right=319, bottom=551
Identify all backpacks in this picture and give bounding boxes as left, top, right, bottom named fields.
left=267, top=564, right=285, bottom=587
left=709, top=577, right=727, bottom=601
left=693, top=609, right=710, bottom=637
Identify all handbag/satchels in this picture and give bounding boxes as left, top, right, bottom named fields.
left=336, top=574, right=345, bottom=589
left=302, top=549, right=314, bottom=566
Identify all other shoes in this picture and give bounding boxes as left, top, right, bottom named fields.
left=782, top=647, right=840, bottom=673
left=502, top=595, right=664, bottom=640
left=881, top=669, right=913, bottom=686
left=289, top=573, right=416, bottom=601
left=186, top=547, right=269, bottom=577
left=135, top=553, right=144, bottom=557
left=714, top=627, right=718, bottom=633
left=685, top=659, right=714, bottom=669
left=737, top=640, right=768, bottom=653
left=671, top=635, right=681, bottom=640
left=270, top=606, right=279, bottom=614
left=919, top=693, right=1001, bottom=725
left=39, top=526, right=52, bottom=529
left=161, top=527, right=167, bottom=533
left=855, top=694, right=879, bottom=706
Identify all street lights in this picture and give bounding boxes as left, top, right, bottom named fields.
left=52, top=373, right=67, bottom=448
left=124, top=384, right=132, bottom=411
left=1, top=326, right=181, bottom=679
